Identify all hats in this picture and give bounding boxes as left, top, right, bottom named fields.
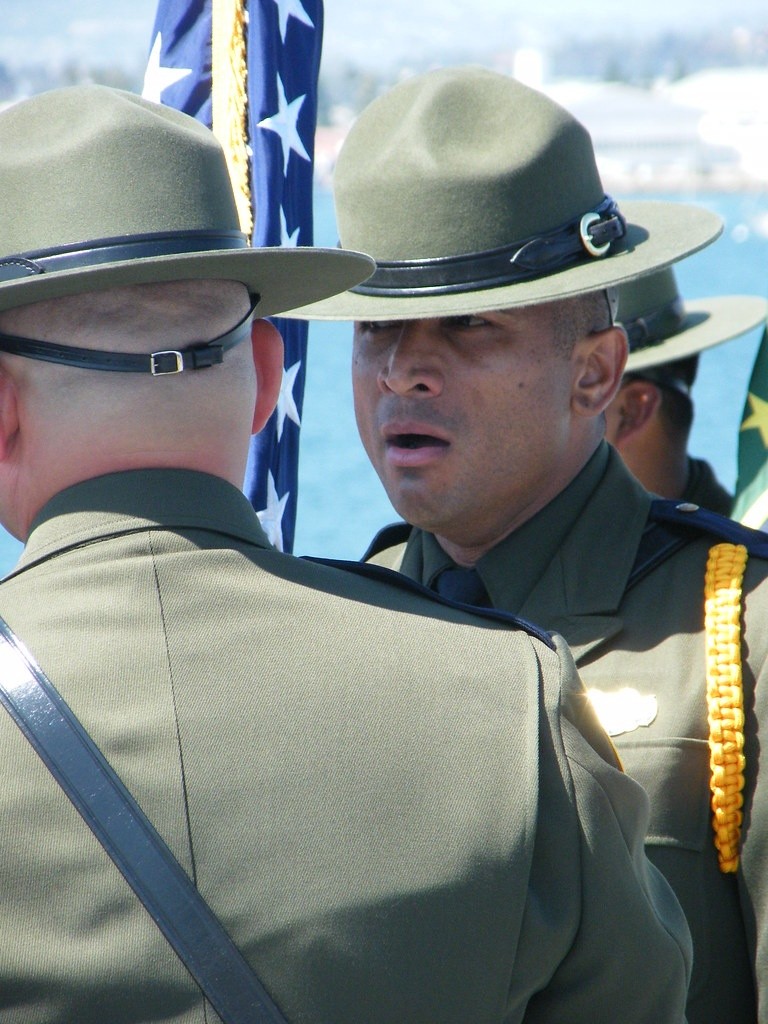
left=614, top=265, right=768, bottom=374
left=0, top=85, right=376, bottom=320
left=267, top=66, right=724, bottom=320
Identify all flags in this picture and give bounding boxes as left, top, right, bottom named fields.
left=142, top=0, right=329, bottom=560
left=732, top=319, right=768, bottom=537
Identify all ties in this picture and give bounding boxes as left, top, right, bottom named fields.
left=434, top=568, right=488, bottom=606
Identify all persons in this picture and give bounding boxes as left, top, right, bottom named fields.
left=0, top=86, right=698, bottom=1024
left=263, top=65, right=768, bottom=1024
left=605, top=260, right=765, bottom=515
